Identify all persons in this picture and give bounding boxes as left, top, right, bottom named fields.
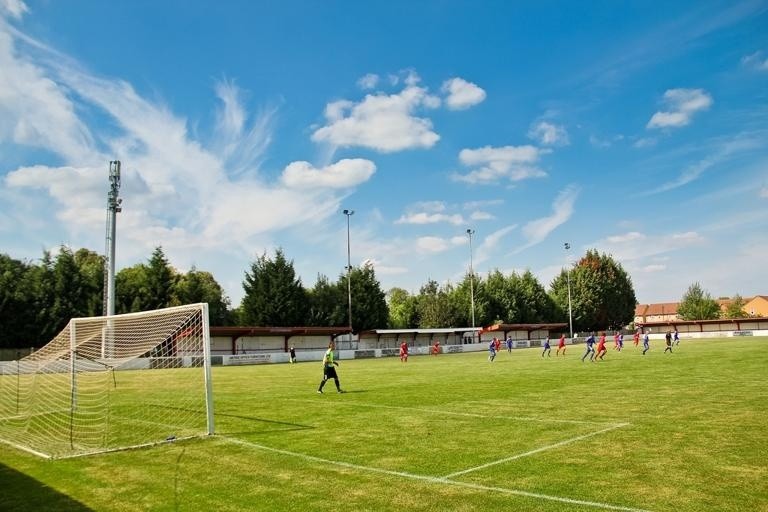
left=488, top=335, right=514, bottom=363
left=317, top=340, right=345, bottom=394
left=540, top=327, right=681, bottom=363
left=468, top=336, right=471, bottom=344
left=464, top=336, right=467, bottom=344
left=431, top=335, right=440, bottom=356
left=400, top=337, right=409, bottom=363
left=290, top=342, right=296, bottom=365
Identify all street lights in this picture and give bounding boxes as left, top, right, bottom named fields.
left=467, top=229, right=474, bottom=344
left=564, top=243, right=574, bottom=338
left=103, top=161, right=122, bottom=358
left=344, top=209, right=354, bottom=350
